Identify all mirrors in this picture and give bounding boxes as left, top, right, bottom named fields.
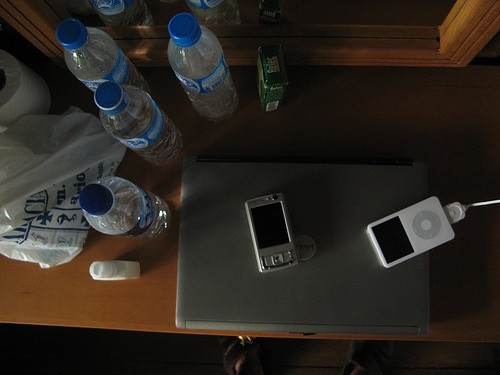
left=0, top=0, right=500, bottom=68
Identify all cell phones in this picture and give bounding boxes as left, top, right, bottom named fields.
left=244, top=193, right=298, bottom=273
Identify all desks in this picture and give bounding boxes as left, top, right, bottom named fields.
left=0, top=29, right=500, bottom=342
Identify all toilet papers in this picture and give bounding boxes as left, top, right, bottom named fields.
left=0, top=50, right=52, bottom=127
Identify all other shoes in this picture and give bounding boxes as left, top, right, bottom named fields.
left=342, top=341, right=392, bottom=375
left=221, top=334, right=260, bottom=375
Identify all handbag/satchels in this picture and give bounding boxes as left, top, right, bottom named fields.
left=0, top=104, right=127, bottom=269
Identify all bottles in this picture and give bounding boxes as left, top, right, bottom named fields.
left=78, top=176, right=171, bottom=240
left=167, top=12, right=240, bottom=122
left=89, top=0, right=153, bottom=26
left=89, top=260, right=141, bottom=281
left=184, top=0, right=241, bottom=25
left=55, top=17, right=152, bottom=95
left=94, top=80, right=184, bottom=167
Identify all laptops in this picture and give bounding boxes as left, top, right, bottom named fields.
left=175, top=155, right=432, bottom=337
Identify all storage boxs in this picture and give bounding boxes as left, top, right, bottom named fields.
left=257, top=43, right=290, bottom=113
left=256, top=0, right=284, bottom=25
